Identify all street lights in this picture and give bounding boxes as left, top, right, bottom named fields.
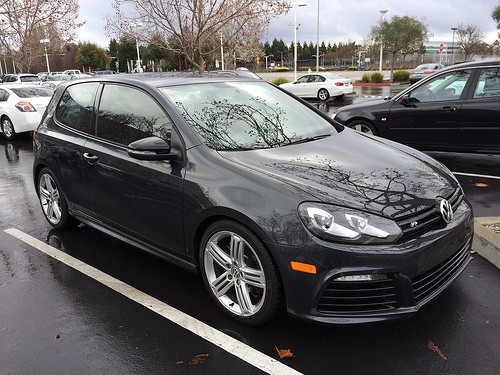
left=129, top=22, right=143, bottom=72
left=277, top=51, right=282, bottom=66
left=450, top=28, right=457, bottom=64
left=293, top=3, right=306, bottom=81
left=379, top=9, right=388, bottom=71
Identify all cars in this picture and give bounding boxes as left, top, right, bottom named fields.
left=331, top=57, right=500, bottom=156
left=279, top=71, right=355, bottom=101
left=424, top=71, right=499, bottom=98
left=0, top=69, right=117, bottom=89
left=408, top=63, right=445, bottom=85
left=0, top=84, right=64, bottom=140
left=33, top=69, right=476, bottom=329
